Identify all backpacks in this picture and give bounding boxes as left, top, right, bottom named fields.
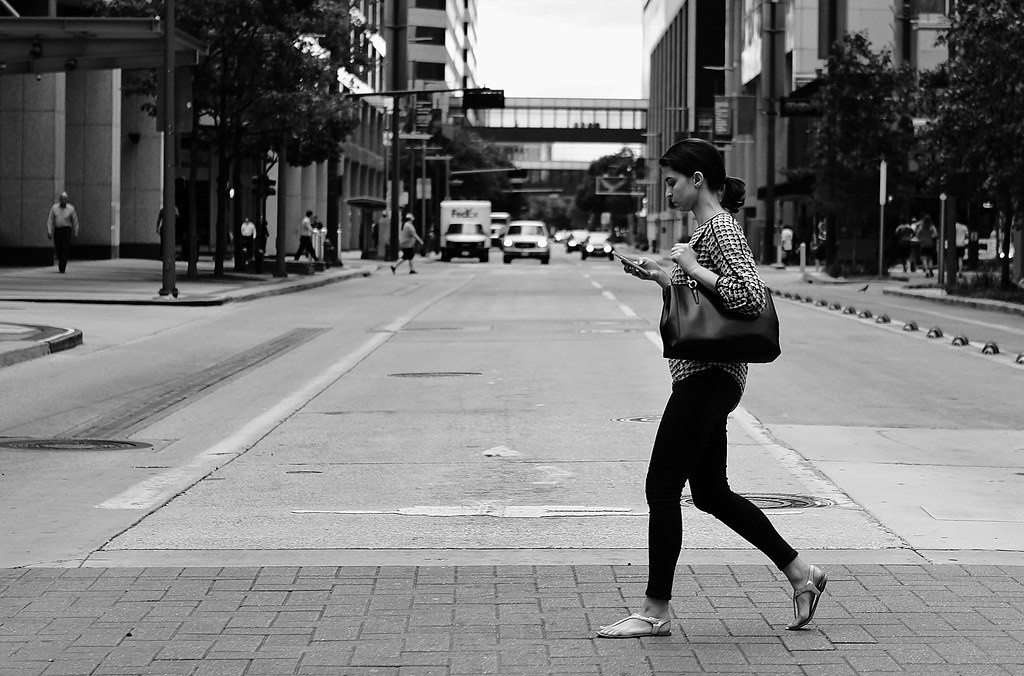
left=919, top=225, right=934, bottom=249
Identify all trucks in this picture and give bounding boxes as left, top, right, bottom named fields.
left=441, top=199, right=491, bottom=261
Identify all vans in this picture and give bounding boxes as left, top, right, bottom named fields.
left=962, top=238, right=999, bottom=264
left=490, top=211, right=511, bottom=249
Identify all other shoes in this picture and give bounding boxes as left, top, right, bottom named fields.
left=903, top=266, right=907, bottom=271
left=926, top=273, right=929, bottom=278
left=929, top=269, right=934, bottom=278
left=409, top=270, right=416, bottom=274
left=959, top=273, right=963, bottom=278
left=390, top=265, right=395, bottom=273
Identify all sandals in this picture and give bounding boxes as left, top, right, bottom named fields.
left=787, top=565, right=827, bottom=631
left=596, top=612, right=672, bottom=638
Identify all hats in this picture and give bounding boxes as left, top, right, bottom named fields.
left=406, top=213, right=415, bottom=221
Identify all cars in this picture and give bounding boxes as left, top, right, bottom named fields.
left=502, top=220, right=554, bottom=264
left=581, top=236, right=615, bottom=261
left=554, top=230, right=571, bottom=242
left=567, top=232, right=588, bottom=253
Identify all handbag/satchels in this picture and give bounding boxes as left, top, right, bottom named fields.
left=659, top=213, right=781, bottom=364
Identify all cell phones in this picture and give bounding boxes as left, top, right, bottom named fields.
left=612, top=251, right=650, bottom=276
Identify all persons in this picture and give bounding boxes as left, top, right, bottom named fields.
left=242, top=217, right=269, bottom=263
left=896, top=213, right=970, bottom=279
left=371, top=210, right=439, bottom=275
left=47, top=191, right=79, bottom=273
left=779, top=225, right=794, bottom=265
left=156, top=205, right=179, bottom=258
left=295, top=210, right=323, bottom=263
left=596, top=139, right=828, bottom=637
